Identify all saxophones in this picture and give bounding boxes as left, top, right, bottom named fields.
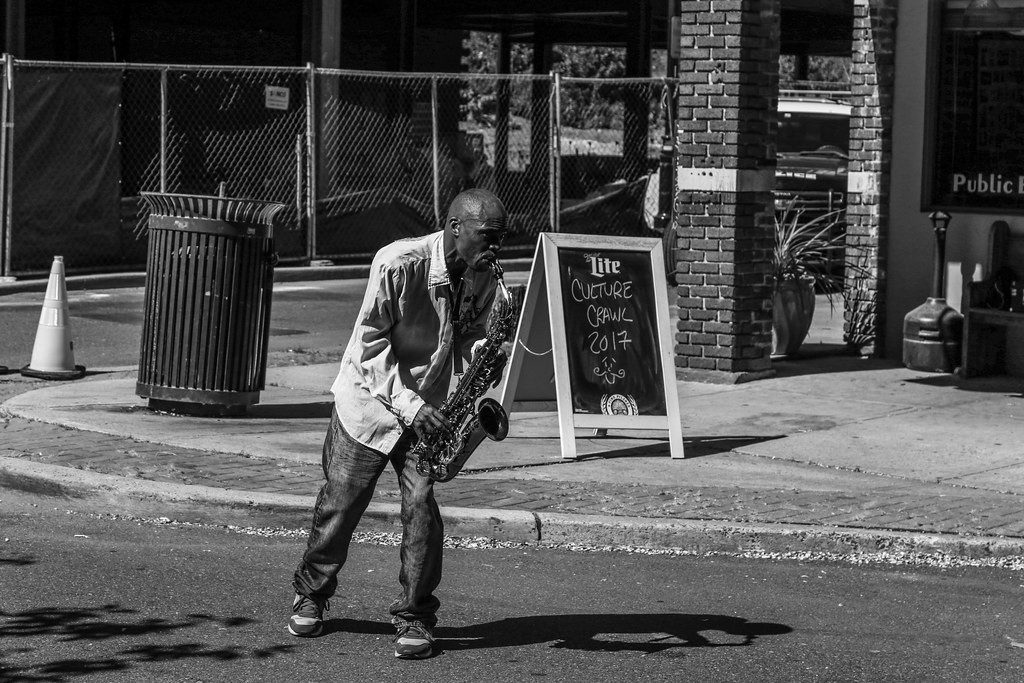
left=411, top=257, right=518, bottom=482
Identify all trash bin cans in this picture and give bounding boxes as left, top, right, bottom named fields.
left=134, top=190, right=289, bottom=417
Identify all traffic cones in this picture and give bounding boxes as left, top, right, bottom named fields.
left=17, top=254, right=86, bottom=380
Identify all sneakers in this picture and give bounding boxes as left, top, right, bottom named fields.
left=288, top=594, right=330, bottom=636
left=392, top=620, right=435, bottom=659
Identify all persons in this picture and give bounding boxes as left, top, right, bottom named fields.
left=287, top=188, right=508, bottom=660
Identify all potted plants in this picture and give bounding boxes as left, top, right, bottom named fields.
left=772, top=195, right=879, bottom=355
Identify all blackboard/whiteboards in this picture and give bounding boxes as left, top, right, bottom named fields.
left=542, top=233, right=683, bottom=429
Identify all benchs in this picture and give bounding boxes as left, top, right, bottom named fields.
left=954, top=221, right=1024, bottom=379
